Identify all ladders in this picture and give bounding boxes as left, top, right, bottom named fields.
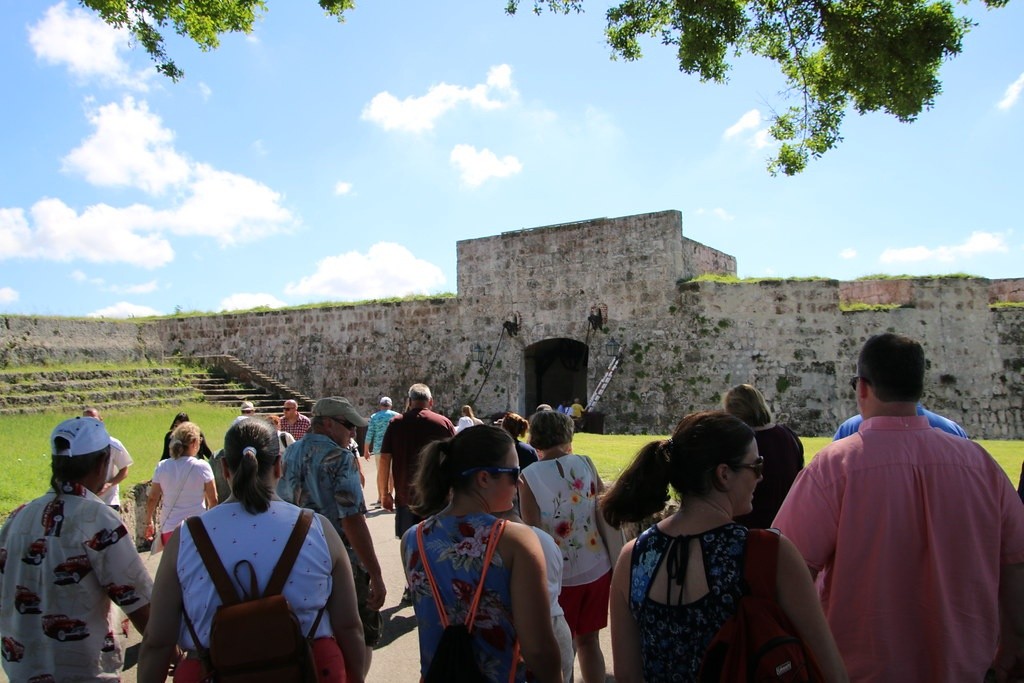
left=584, top=343, right=626, bottom=413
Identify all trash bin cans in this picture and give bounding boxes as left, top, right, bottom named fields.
left=581, top=411, right=606, bottom=434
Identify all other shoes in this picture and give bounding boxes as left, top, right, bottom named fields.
left=376, top=501, right=381, bottom=508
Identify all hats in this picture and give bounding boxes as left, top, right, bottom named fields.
left=312, top=396, right=368, bottom=428
left=51, top=416, right=123, bottom=456
left=241, top=402, right=254, bottom=410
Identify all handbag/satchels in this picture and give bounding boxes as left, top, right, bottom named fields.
left=150, top=531, right=164, bottom=555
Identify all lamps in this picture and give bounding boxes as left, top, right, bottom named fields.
left=606, top=336, right=624, bottom=360
left=471, top=342, right=491, bottom=369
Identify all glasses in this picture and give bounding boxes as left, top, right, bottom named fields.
left=199, top=437, right=202, bottom=443
left=707, top=456, right=764, bottom=478
left=462, top=466, right=520, bottom=485
left=849, top=377, right=871, bottom=391
left=242, top=411, right=251, bottom=414
left=330, top=416, right=355, bottom=431
left=283, top=407, right=294, bottom=411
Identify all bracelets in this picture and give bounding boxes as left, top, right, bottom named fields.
left=372, top=573, right=380, bottom=579
left=177, top=649, right=184, bottom=661
left=143, top=521, right=153, bottom=528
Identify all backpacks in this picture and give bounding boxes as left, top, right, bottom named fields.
left=181, top=509, right=328, bottom=683
left=698, top=529, right=823, bottom=683
left=417, top=517, right=520, bottom=683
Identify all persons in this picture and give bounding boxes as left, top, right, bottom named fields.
left=456, top=406, right=476, bottom=433
left=347, top=438, right=365, bottom=488
left=502, top=412, right=539, bottom=471
left=378, top=383, right=458, bottom=602
left=265, top=415, right=296, bottom=454
left=240, top=401, right=256, bottom=417
left=280, top=399, right=311, bottom=441
left=527, top=404, right=572, bottom=460
left=400, top=424, right=563, bottom=683
left=0, top=416, right=186, bottom=683
left=517, top=411, right=623, bottom=683
left=145, top=421, right=218, bottom=548
left=276, top=396, right=387, bottom=680
left=771, top=333, right=1024, bottom=683
left=137, top=417, right=366, bottom=683
left=601, top=410, right=849, bottom=683
left=161, top=412, right=212, bottom=461
left=365, top=397, right=399, bottom=508
left=83, top=408, right=133, bottom=511
left=831, top=402, right=967, bottom=442
left=558, top=398, right=584, bottom=417
left=725, top=384, right=804, bottom=529
